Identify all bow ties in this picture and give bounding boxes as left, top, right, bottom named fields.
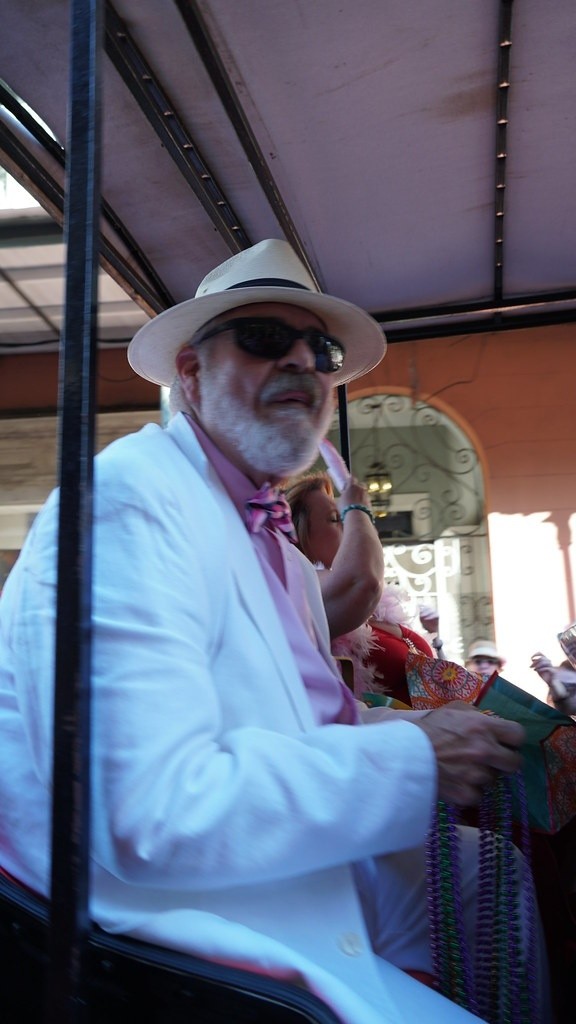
left=242, top=484, right=299, bottom=541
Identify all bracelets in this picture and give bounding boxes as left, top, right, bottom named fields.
left=340, top=504, right=374, bottom=525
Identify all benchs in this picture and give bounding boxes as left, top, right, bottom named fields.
left=0, top=865, right=344, bottom=1024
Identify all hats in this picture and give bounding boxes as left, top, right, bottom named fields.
left=128, top=239, right=387, bottom=388
left=463, top=639, right=507, bottom=668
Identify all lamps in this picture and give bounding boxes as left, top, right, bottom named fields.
left=362, top=461, right=394, bottom=522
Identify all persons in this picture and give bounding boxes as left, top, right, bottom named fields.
left=421, top=631, right=576, bottom=724
left=280, top=468, right=576, bottom=1024
left=2, top=232, right=548, bottom=1024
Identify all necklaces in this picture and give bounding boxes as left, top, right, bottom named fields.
left=422, top=763, right=543, bottom=1023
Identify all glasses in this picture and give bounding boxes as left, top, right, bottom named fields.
left=193, top=316, right=347, bottom=376
